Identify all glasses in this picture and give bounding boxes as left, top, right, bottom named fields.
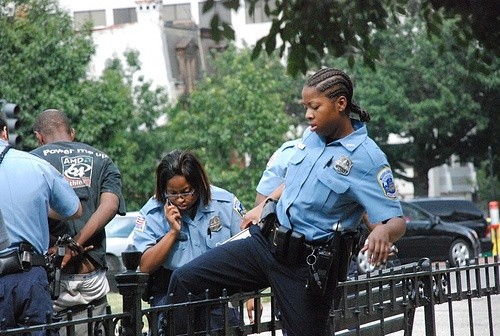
left=163, top=187, right=197, bottom=200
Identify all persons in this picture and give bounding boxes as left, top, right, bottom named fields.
left=0, top=112, right=82, bottom=336
left=163, top=66, right=406, bottom=336
left=256, top=137, right=399, bottom=256
left=121, top=149, right=263, bottom=336
left=27, top=109, right=126, bottom=336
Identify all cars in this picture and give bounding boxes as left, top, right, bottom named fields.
left=401, top=200, right=494, bottom=252
left=397, top=200, right=481, bottom=268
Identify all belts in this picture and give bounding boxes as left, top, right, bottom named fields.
left=31, top=256, right=50, bottom=266
left=266, top=223, right=332, bottom=269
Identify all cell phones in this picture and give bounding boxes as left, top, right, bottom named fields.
left=166, top=201, right=183, bottom=217
